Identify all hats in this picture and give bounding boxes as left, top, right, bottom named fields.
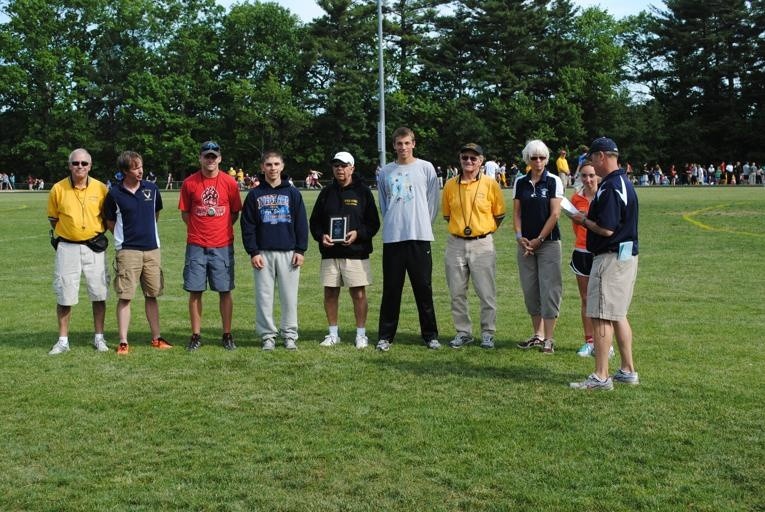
left=331, top=151, right=355, bottom=168
left=586, top=137, right=618, bottom=157
left=200, top=140, right=220, bottom=158
left=461, top=142, right=483, bottom=156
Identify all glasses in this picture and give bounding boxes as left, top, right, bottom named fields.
left=334, top=165, right=347, bottom=167
left=530, top=155, right=546, bottom=161
left=70, top=161, right=89, bottom=166
left=461, top=156, right=477, bottom=161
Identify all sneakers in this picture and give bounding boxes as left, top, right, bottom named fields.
left=320, top=333, right=341, bottom=346
left=448, top=335, right=475, bottom=348
left=261, top=338, right=276, bottom=350
left=376, top=339, right=391, bottom=351
left=283, top=338, right=297, bottom=349
left=516, top=336, right=544, bottom=349
left=569, top=372, right=614, bottom=392
left=49, top=336, right=70, bottom=354
left=592, top=345, right=614, bottom=358
left=150, top=337, right=172, bottom=350
left=542, top=337, right=554, bottom=355
left=187, top=333, right=202, bottom=351
left=480, top=333, right=495, bottom=348
left=612, top=370, right=638, bottom=386
left=94, top=333, right=108, bottom=352
left=576, top=342, right=594, bottom=358
left=222, top=333, right=236, bottom=350
left=354, top=334, right=368, bottom=349
left=424, top=338, right=441, bottom=350
left=118, top=342, right=129, bottom=355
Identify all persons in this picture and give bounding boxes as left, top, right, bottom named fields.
left=570, top=161, right=615, bottom=358
left=28, top=175, right=33, bottom=190
left=10, top=173, right=15, bottom=189
left=103, top=151, right=173, bottom=354
left=305, top=169, right=323, bottom=190
left=681, top=161, right=765, bottom=185
left=0, top=174, right=4, bottom=190
left=106, top=179, right=111, bottom=187
left=671, top=164, right=680, bottom=187
left=632, top=174, right=639, bottom=185
left=627, top=160, right=632, bottom=180
left=165, top=173, right=173, bottom=190
left=556, top=151, right=570, bottom=195
left=289, top=177, right=295, bottom=188
left=3, top=173, right=13, bottom=190
left=376, top=165, right=379, bottom=189
left=240, top=150, right=308, bottom=350
left=436, top=166, right=443, bottom=190
left=33, top=179, right=39, bottom=190
left=48, top=149, right=109, bottom=356
left=480, top=156, right=507, bottom=188
left=442, top=143, right=506, bottom=348
left=446, top=165, right=458, bottom=180
left=227, top=166, right=236, bottom=179
left=573, top=145, right=589, bottom=190
left=509, top=162, right=518, bottom=186
left=146, top=171, right=157, bottom=184
left=309, top=152, right=380, bottom=349
left=237, top=168, right=243, bottom=190
left=512, top=140, right=564, bottom=355
left=115, top=172, right=124, bottom=183
left=38, top=179, right=44, bottom=190
left=638, top=162, right=670, bottom=186
left=179, top=141, right=243, bottom=352
left=517, top=170, right=522, bottom=177
left=244, top=173, right=260, bottom=190
left=567, top=138, right=639, bottom=390
left=377, top=127, right=441, bottom=352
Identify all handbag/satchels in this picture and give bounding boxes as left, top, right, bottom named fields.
left=88, top=233, right=109, bottom=253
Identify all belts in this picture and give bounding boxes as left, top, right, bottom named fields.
left=451, top=233, right=490, bottom=241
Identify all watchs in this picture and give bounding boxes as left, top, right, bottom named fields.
left=581, top=217, right=586, bottom=227
left=539, top=236, right=545, bottom=243
